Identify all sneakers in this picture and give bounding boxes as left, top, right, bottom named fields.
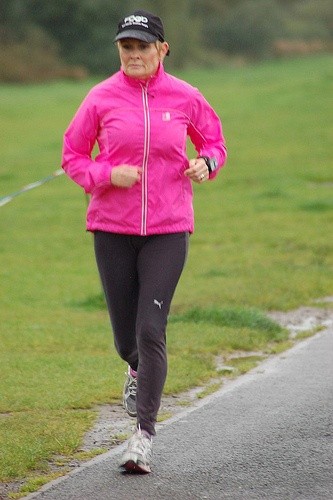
left=123, top=365, right=139, bottom=416
left=116, top=423, right=155, bottom=474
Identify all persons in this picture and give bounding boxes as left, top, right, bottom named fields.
left=62, top=10, right=227, bottom=473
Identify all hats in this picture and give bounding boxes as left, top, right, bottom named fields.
left=113, top=10, right=165, bottom=45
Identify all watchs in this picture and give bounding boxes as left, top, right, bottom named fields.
left=197, top=156, right=215, bottom=173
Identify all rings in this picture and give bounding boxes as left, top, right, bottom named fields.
left=201, top=174, right=205, bottom=179
